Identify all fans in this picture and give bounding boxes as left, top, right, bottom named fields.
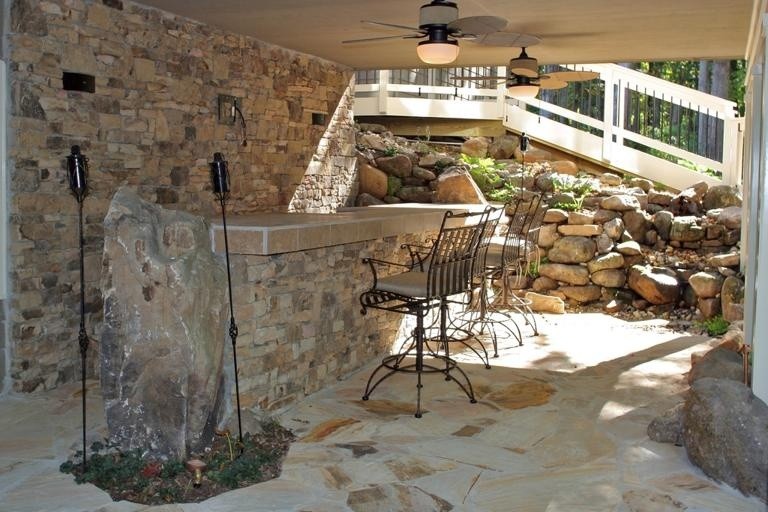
left=342, top=0, right=543, bottom=65
left=450, top=45, right=599, bottom=102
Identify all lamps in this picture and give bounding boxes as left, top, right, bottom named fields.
left=416, top=39, right=460, bottom=65
left=507, top=77, right=541, bottom=100
